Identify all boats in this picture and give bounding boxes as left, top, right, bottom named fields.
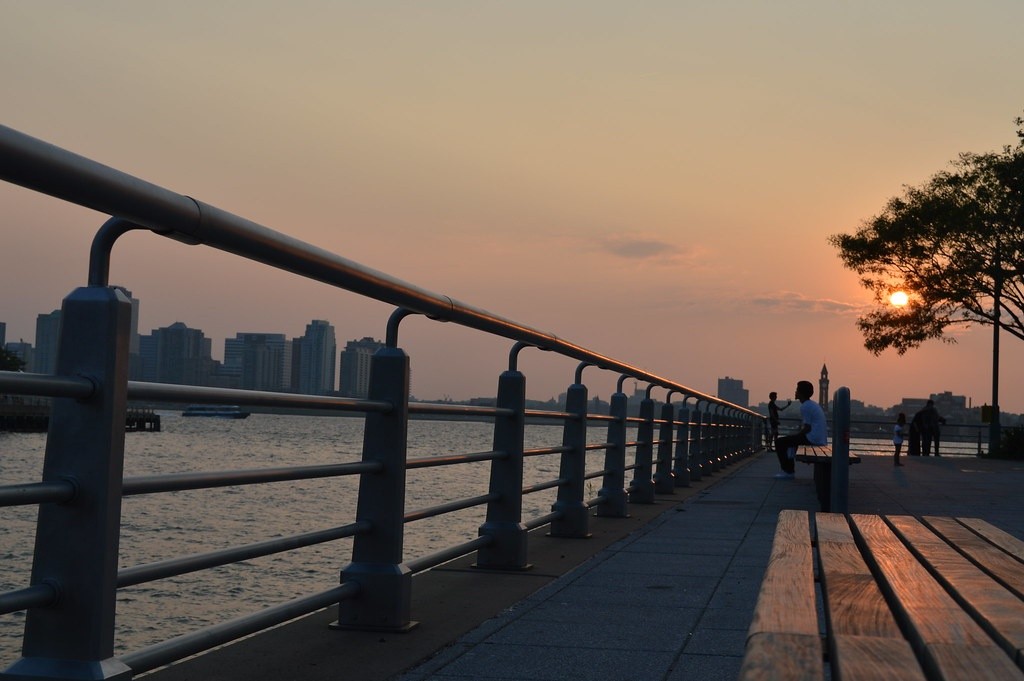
left=181, top=403, right=252, bottom=419
left=1, top=392, right=161, bottom=432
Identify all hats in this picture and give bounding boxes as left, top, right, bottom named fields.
left=898, top=413, right=906, bottom=422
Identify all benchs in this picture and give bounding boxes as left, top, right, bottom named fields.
left=797, top=441, right=861, bottom=505
left=742, top=501, right=1024, bottom=681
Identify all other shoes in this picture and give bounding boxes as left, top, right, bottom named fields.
left=895, top=463, right=905, bottom=466
left=776, top=470, right=795, bottom=479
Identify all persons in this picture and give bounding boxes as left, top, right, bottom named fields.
left=768, top=392, right=792, bottom=450
left=921, top=400, right=944, bottom=457
left=908, top=405, right=931, bottom=457
left=894, top=417, right=909, bottom=466
left=775, top=381, right=828, bottom=479
